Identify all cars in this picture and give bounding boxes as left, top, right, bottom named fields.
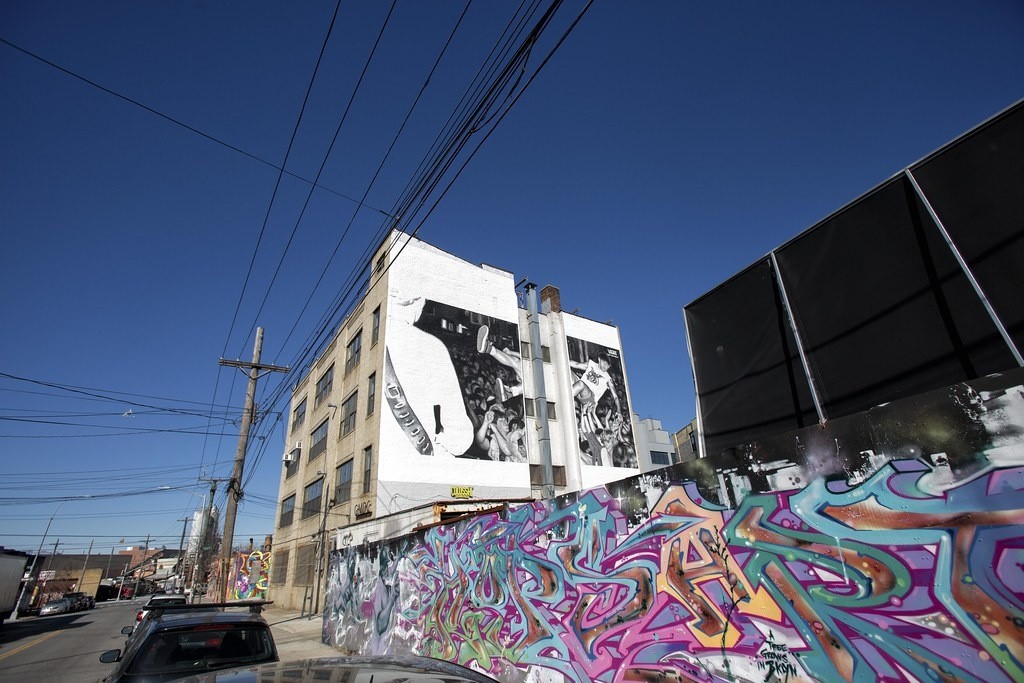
left=165, top=583, right=209, bottom=595
left=159, top=653, right=500, bottom=683
left=39, top=591, right=95, bottom=617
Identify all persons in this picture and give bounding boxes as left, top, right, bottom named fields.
left=380, top=291, right=638, bottom=476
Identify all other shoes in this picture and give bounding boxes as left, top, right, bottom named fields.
left=496, top=378, right=513, bottom=402
left=476, top=325, right=489, bottom=354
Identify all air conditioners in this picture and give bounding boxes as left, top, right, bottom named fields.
left=282, top=453, right=291, bottom=460
left=296, top=441, right=301, bottom=449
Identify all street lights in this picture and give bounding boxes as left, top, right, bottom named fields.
left=156, top=484, right=207, bottom=604
left=8, top=494, right=94, bottom=620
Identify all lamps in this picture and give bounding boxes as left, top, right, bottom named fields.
left=327, top=403, right=337, bottom=408
left=317, top=471, right=326, bottom=476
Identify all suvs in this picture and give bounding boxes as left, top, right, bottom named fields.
left=132, top=591, right=191, bottom=635
left=98, top=599, right=279, bottom=683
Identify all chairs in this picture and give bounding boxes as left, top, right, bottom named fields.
left=220, top=630, right=253, bottom=658
left=152, top=632, right=183, bottom=664
